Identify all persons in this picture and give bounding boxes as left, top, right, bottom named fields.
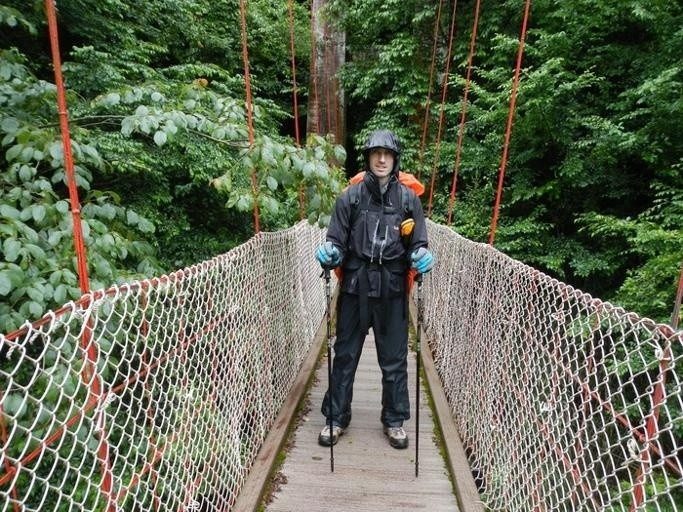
left=315, top=128, right=435, bottom=450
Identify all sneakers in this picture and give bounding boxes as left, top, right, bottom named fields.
left=382, top=425, right=408, bottom=449
left=319, top=425, right=344, bottom=447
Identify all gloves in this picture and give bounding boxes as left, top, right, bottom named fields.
left=314, top=242, right=341, bottom=266
left=411, top=247, right=434, bottom=273
